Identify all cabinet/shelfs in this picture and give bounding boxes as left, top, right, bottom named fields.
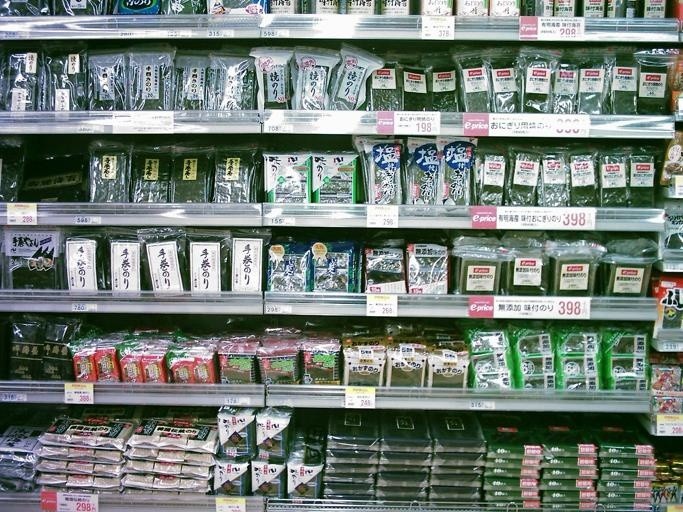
left=0, top=0, right=683, bottom=512
